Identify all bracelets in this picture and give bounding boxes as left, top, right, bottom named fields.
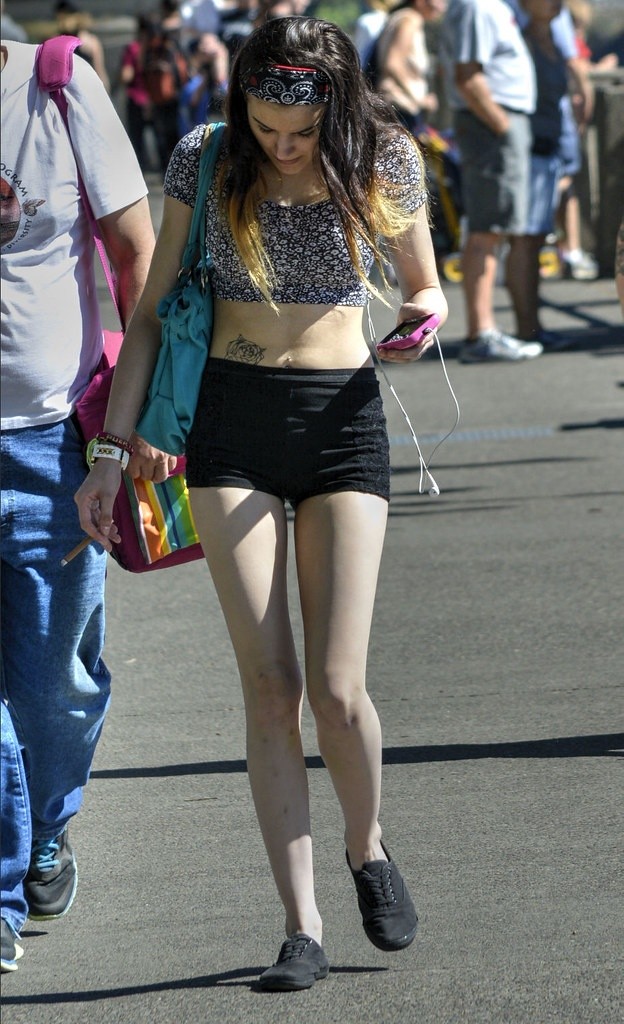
left=93, top=431, right=135, bottom=471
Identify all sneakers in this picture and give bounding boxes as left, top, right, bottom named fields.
left=22, top=827, right=79, bottom=921
left=259, top=933, right=330, bottom=989
left=346, top=840, right=418, bottom=951
left=0, top=917, right=24, bottom=972
left=459, top=330, right=543, bottom=363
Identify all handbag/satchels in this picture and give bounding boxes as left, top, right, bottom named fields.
left=75, top=329, right=206, bottom=574
left=133, top=121, right=228, bottom=456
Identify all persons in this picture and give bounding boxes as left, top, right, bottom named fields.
left=518, top=0, right=624, bottom=282
left=0, top=0, right=317, bottom=187
left=0, top=35, right=156, bottom=972
left=355, top=0, right=402, bottom=72
left=362, top=0, right=447, bottom=140
left=439, top=0, right=544, bottom=365
left=73, top=16, right=449, bottom=993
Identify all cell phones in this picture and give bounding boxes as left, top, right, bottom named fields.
left=377, top=313, right=440, bottom=349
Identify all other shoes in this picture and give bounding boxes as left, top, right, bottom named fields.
left=562, top=248, right=599, bottom=281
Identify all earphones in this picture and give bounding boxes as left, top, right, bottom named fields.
left=429, top=474, right=440, bottom=497
